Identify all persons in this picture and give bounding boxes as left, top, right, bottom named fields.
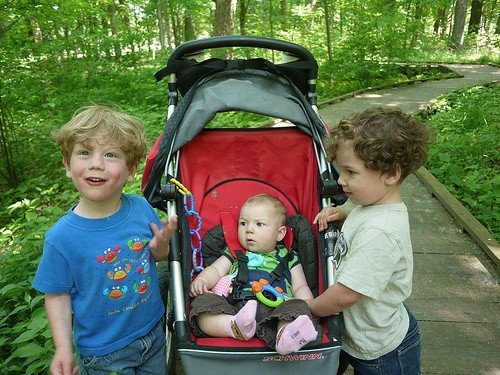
left=189, top=193, right=319, bottom=356
left=32, top=102, right=177, bottom=375
left=303, top=105, right=436, bottom=375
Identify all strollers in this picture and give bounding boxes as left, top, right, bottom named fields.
left=138, top=34, right=350, bottom=375
